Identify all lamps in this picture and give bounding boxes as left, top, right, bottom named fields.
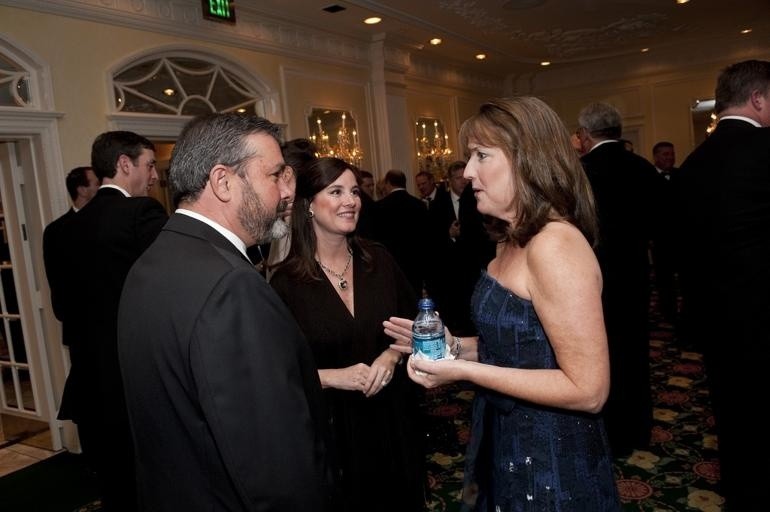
left=705, top=110, right=720, bottom=137
left=414, top=118, right=454, bottom=180
left=307, top=109, right=365, bottom=173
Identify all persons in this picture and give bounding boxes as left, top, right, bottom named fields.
left=435, top=162, right=483, bottom=338
left=382, top=95, right=622, bottom=512
left=667, top=61, right=769, bottom=512
left=458, top=180, right=495, bottom=336
left=355, top=171, right=375, bottom=199
left=375, top=170, right=427, bottom=320
left=42, top=166, right=98, bottom=349
left=375, top=179, right=388, bottom=200
left=56, top=131, right=171, bottom=512
left=267, top=159, right=425, bottom=512
left=652, top=141, right=679, bottom=182
left=567, top=103, right=666, bottom=454
left=252, top=138, right=318, bottom=281
left=415, top=172, right=449, bottom=236
left=118, top=114, right=331, bottom=510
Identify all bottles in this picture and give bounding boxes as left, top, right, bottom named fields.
left=411, top=299, right=446, bottom=362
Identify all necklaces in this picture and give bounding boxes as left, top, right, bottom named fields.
left=312, top=250, right=353, bottom=290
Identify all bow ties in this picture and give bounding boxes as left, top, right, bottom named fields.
left=661, top=172, right=672, bottom=177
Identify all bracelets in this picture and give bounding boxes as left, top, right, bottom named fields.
left=454, top=337, right=461, bottom=360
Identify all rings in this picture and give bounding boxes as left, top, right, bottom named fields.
left=381, top=381, right=387, bottom=387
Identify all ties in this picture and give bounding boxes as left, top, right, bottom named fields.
left=427, top=198, right=431, bottom=209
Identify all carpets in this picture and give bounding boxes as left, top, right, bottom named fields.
left=0, top=449, right=100, bottom=512
left=426, top=281, right=729, bottom=509
